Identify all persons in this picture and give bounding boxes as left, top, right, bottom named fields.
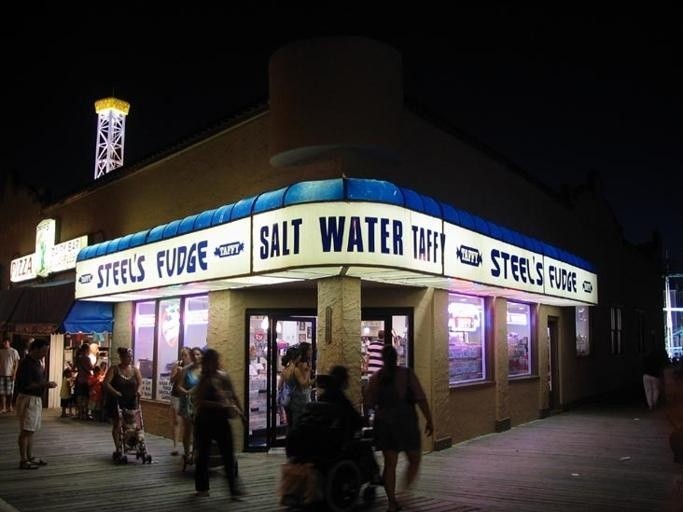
left=170, top=347, right=250, bottom=498
left=278, top=330, right=434, bottom=512
left=641, top=352, right=662, bottom=410
left=1, top=337, right=143, bottom=470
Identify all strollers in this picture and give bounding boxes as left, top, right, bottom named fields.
left=112, top=392, right=152, bottom=466
left=181, top=387, right=243, bottom=480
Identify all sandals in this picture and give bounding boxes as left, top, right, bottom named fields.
left=19, top=458, right=47, bottom=470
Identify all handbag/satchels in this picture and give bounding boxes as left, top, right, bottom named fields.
left=278, top=382, right=296, bottom=406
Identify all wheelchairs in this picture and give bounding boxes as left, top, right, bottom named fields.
left=278, top=401, right=385, bottom=511
